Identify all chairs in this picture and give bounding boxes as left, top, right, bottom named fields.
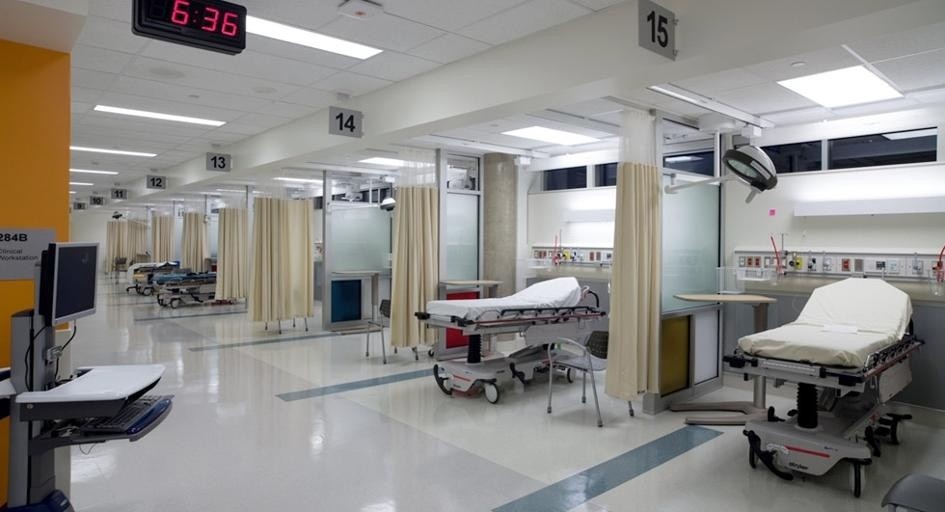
left=111, top=258, right=127, bottom=284
left=366, top=300, right=419, bottom=364
left=547, top=331, right=634, bottom=427
left=881, top=473, right=945, bottom=512
left=265, top=317, right=308, bottom=334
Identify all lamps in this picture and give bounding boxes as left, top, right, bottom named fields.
left=666, top=145, right=777, bottom=203
left=112, top=211, right=122, bottom=219
left=380, top=194, right=396, bottom=212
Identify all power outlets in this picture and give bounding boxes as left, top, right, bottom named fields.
left=887, top=260, right=900, bottom=273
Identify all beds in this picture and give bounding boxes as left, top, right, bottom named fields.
left=415, top=276, right=606, bottom=403
left=126, top=262, right=177, bottom=296
left=721, top=279, right=924, bottom=497
left=151, top=272, right=216, bottom=309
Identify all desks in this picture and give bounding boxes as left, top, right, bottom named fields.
left=329, top=272, right=378, bottom=336
left=673, top=295, right=777, bottom=425
left=439, top=280, right=504, bottom=360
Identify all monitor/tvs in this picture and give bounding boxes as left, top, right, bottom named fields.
left=39, top=241, right=101, bottom=329
